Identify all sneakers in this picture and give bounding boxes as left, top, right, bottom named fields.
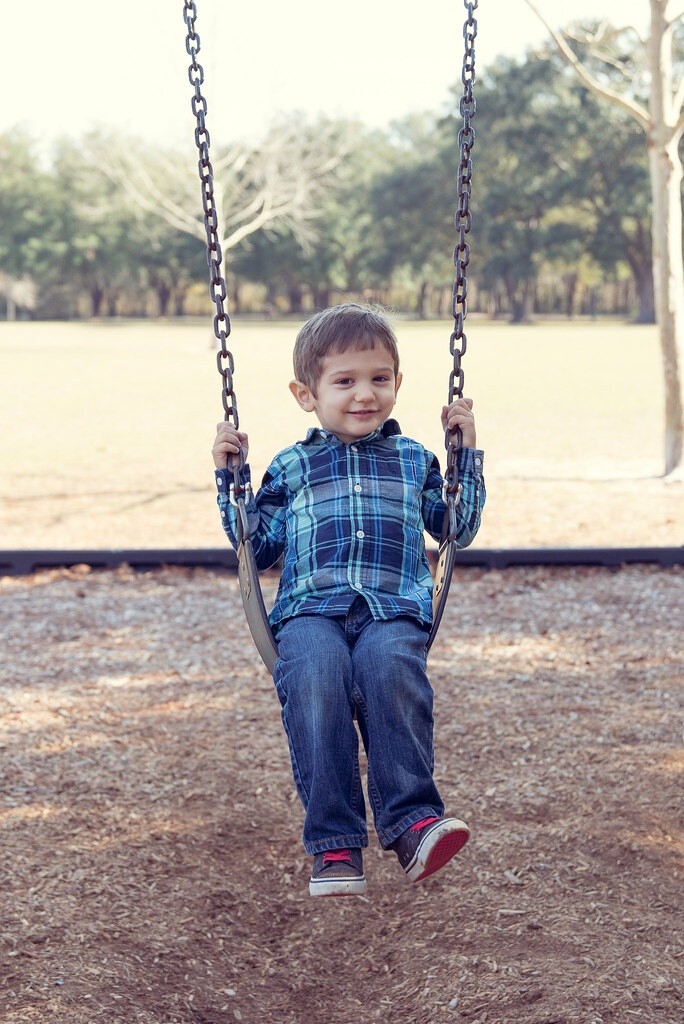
left=394, top=816, right=471, bottom=883
left=309, top=848, right=367, bottom=897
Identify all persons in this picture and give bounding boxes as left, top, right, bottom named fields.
left=209, top=300, right=487, bottom=897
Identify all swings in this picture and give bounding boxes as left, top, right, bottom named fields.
left=182, top=1, right=480, bottom=680
